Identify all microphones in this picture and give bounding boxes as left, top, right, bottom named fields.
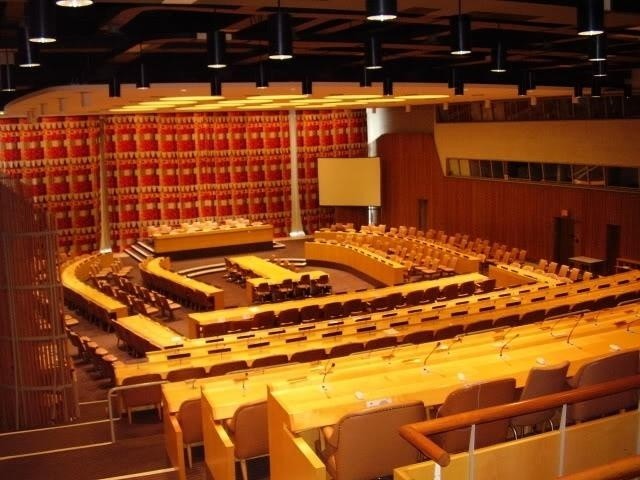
left=131, top=287, right=639, bottom=394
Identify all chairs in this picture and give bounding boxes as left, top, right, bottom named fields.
left=28, top=217, right=640, bottom=480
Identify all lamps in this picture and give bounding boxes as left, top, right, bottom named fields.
left=517, top=59, right=537, bottom=97
left=591, top=83, right=600, bottom=97
left=1, top=39, right=16, bottom=92
left=447, top=59, right=465, bottom=95
left=576, top=2, right=604, bottom=36
left=135, top=39, right=152, bottom=90
left=206, top=5, right=227, bottom=69
left=365, top=29, right=382, bottom=69
left=490, top=21, right=507, bottom=73
left=365, top=2, right=398, bottom=21
left=448, top=1, right=471, bottom=55
left=574, top=86, right=583, bottom=99
left=26, top=2, right=57, bottom=43
left=55, top=1, right=94, bottom=8
left=383, top=67, right=393, bottom=95
left=18, top=23, right=41, bottom=68
left=593, top=60, right=607, bottom=77
left=267, top=1, right=293, bottom=60
left=255, top=30, right=270, bottom=89
left=359, top=65, right=372, bottom=88
left=210, top=74, right=222, bottom=97
left=108, top=52, right=121, bottom=98
left=589, top=32, right=606, bottom=61
left=302, top=73, right=312, bottom=95
